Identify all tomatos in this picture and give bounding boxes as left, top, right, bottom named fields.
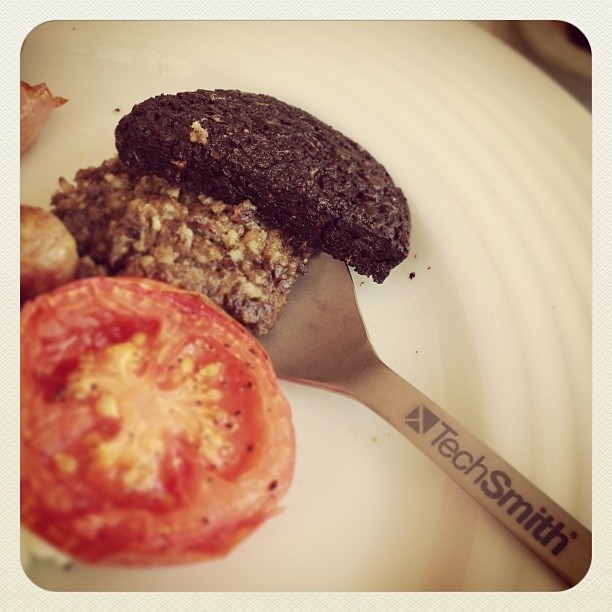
left=19, top=280, right=295, bottom=565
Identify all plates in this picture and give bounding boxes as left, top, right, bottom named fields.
left=20, top=21, right=592, bottom=591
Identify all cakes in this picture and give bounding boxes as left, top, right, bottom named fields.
left=52, top=153, right=317, bottom=333
left=115, top=89, right=409, bottom=282
